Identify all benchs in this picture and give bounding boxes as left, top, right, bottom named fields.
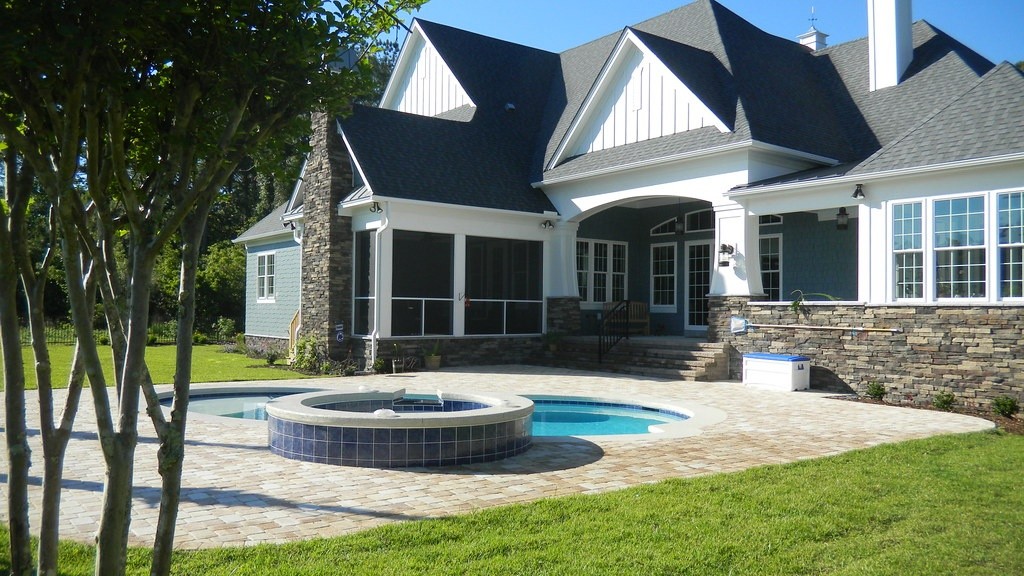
left=602, top=302, right=650, bottom=337
left=742, top=352, right=811, bottom=392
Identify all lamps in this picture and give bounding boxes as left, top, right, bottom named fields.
left=851, top=184, right=865, bottom=200
left=541, top=220, right=554, bottom=230
left=282, top=220, right=296, bottom=231
left=835, top=207, right=850, bottom=230
left=674, top=197, right=685, bottom=235
left=718, top=243, right=737, bottom=267
left=369, top=202, right=383, bottom=214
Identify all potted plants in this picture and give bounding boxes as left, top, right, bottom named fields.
left=421, top=338, right=442, bottom=370
left=343, top=362, right=359, bottom=376
left=391, top=343, right=407, bottom=374
